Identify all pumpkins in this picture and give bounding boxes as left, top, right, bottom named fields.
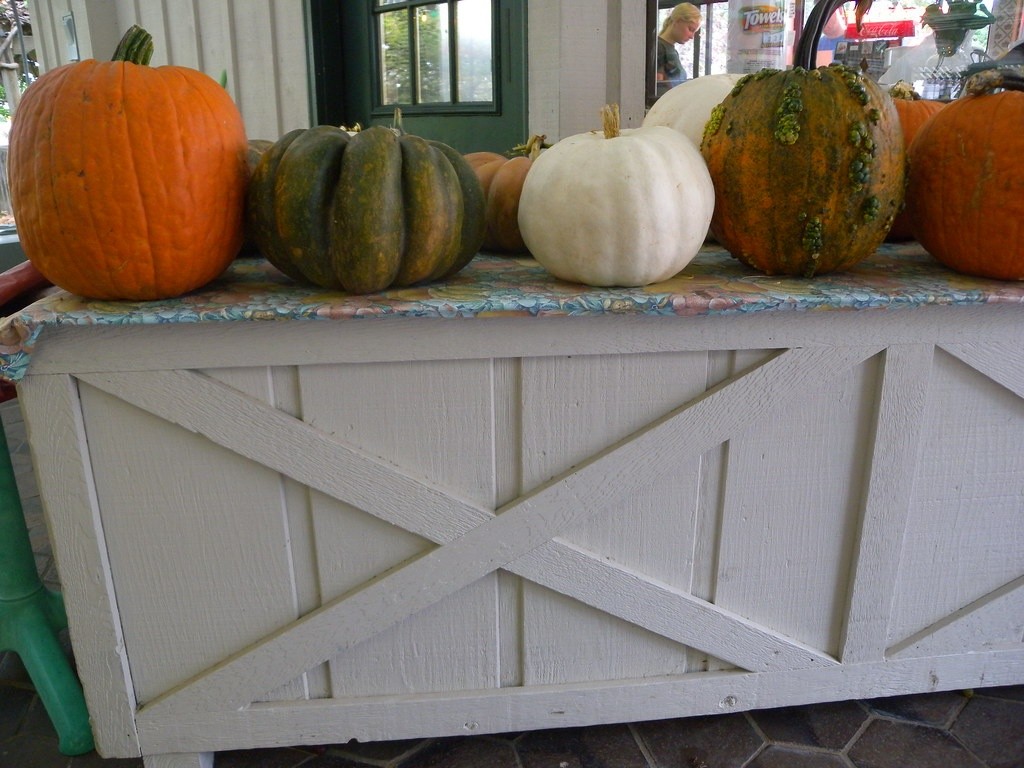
left=7, top=2, right=1023, bottom=297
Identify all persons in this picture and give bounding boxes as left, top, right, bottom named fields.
left=656, top=3, right=702, bottom=81
